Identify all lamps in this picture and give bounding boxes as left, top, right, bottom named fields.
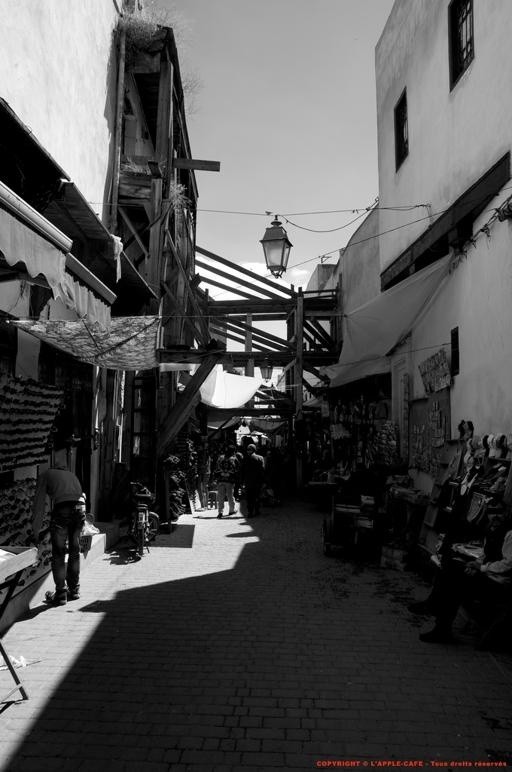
left=259, top=214, right=292, bottom=279
left=260, top=365, right=273, bottom=383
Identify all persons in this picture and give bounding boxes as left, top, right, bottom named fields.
left=31, top=465, right=86, bottom=606
left=407, top=498, right=512, bottom=644
left=214, top=441, right=295, bottom=520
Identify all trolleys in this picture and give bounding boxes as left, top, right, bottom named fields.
left=316, top=469, right=359, bottom=553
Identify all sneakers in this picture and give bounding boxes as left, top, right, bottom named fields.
left=229, top=510, right=237, bottom=515
left=68, top=590, right=80, bottom=600
left=420, top=627, right=452, bottom=641
left=45, top=590, right=67, bottom=604
left=406, top=601, right=436, bottom=617
left=217, top=513, right=223, bottom=518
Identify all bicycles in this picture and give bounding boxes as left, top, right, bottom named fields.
left=126, top=478, right=161, bottom=562
